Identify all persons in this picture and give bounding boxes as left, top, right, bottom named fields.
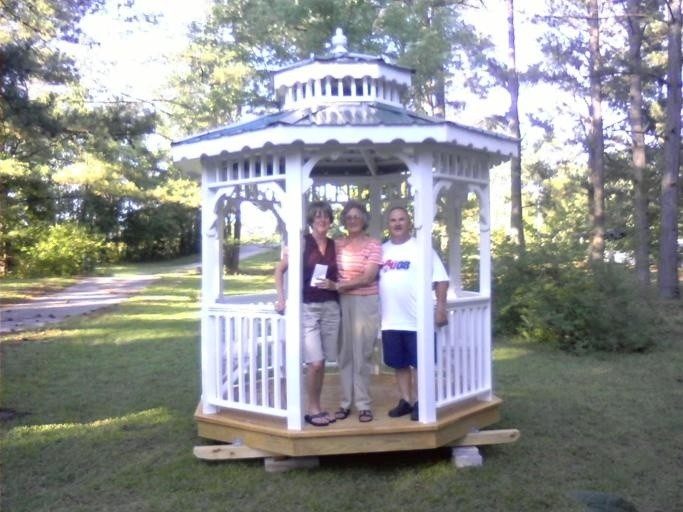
left=274, top=201, right=340, bottom=427
left=373, top=207, right=449, bottom=421
left=283, top=202, right=380, bottom=421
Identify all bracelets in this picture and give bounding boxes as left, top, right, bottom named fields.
left=335, top=282, right=340, bottom=294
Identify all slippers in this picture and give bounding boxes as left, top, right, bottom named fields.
left=305, top=410, right=335, bottom=426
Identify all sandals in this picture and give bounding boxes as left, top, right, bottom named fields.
left=359, top=409, right=373, bottom=422
left=335, top=408, right=350, bottom=419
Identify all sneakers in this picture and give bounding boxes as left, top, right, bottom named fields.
left=410, top=402, right=420, bottom=421
left=389, top=398, right=412, bottom=417
